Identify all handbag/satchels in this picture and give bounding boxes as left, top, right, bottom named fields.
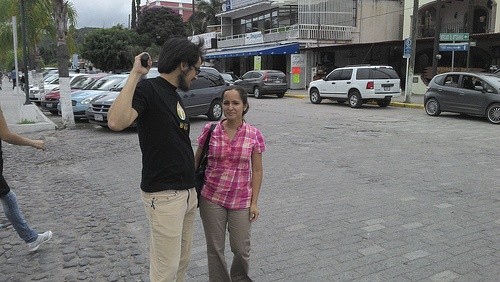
left=193, top=123, right=217, bottom=192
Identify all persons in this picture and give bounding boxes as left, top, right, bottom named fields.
left=0, top=67, right=16, bottom=90
left=0, top=107, right=52, bottom=250
left=108, top=36, right=205, bottom=282
left=195, top=86, right=266, bottom=282
left=89, top=66, right=94, bottom=74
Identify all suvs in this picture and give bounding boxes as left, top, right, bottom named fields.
left=308, top=64, right=402, bottom=108
left=84, top=66, right=229, bottom=128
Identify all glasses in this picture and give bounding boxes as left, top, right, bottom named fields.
left=189, top=62, right=202, bottom=76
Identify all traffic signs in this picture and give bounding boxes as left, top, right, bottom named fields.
left=439, top=33, right=469, bottom=41
left=438, top=42, right=469, bottom=51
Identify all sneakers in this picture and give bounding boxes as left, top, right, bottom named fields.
left=28, top=230, right=53, bottom=251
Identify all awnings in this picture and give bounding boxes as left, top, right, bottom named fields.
left=204, top=43, right=300, bottom=58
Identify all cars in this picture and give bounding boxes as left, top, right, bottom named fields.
left=424, top=72, right=500, bottom=125
left=18, top=67, right=130, bottom=121
left=219, top=72, right=238, bottom=86
left=233, top=69, right=288, bottom=99
